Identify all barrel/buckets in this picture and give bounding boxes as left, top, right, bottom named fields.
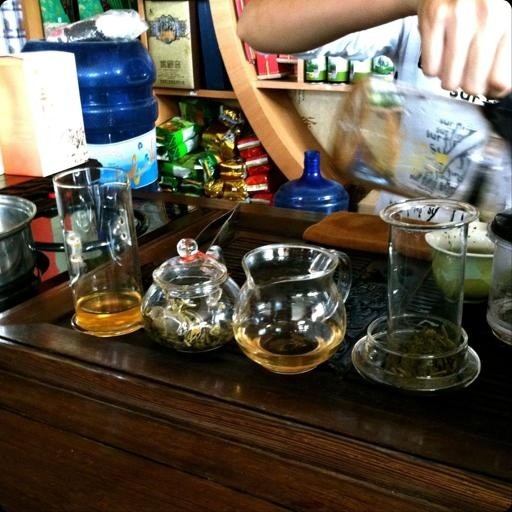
left=18, top=38, right=158, bottom=194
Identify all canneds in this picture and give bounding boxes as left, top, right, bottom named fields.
left=304, top=55, right=395, bottom=84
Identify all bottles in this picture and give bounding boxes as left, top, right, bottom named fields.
left=485, top=211, right=512, bottom=346
left=137, top=236, right=244, bottom=354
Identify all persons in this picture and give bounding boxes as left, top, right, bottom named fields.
left=235, top=1, right=512, bottom=101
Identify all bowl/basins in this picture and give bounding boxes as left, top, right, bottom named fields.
left=420, top=219, right=495, bottom=306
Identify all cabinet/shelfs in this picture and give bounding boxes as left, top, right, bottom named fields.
left=0, top=1, right=511, bottom=249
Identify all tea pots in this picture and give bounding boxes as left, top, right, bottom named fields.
left=228, top=238, right=356, bottom=377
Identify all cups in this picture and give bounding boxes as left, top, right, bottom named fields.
left=378, top=194, right=481, bottom=359
left=327, top=73, right=512, bottom=218
left=51, top=166, right=148, bottom=338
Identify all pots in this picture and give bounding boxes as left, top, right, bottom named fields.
left=1, top=194, right=121, bottom=291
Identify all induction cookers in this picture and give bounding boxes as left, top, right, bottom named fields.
left=0, top=189, right=198, bottom=313
